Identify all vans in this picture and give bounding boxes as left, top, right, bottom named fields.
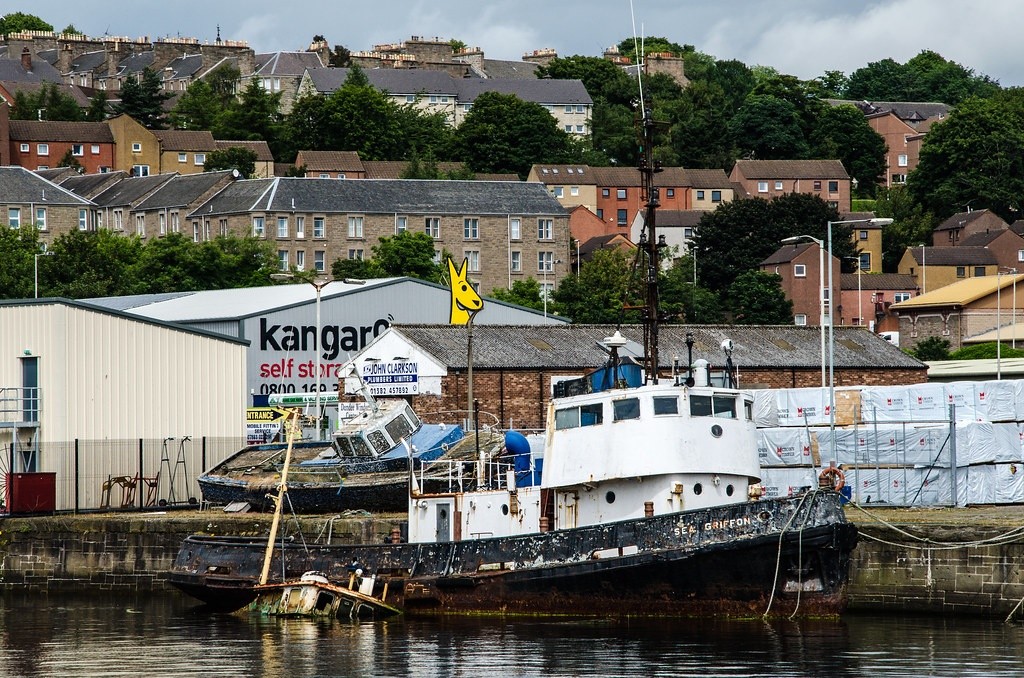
left=876, top=331, right=900, bottom=347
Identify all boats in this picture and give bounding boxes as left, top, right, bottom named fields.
left=170, top=106, right=859, bottom=627
left=254, top=413, right=401, bottom=620
left=196, top=397, right=506, bottom=516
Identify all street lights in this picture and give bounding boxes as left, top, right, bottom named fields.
left=918, top=243, right=925, bottom=293
left=778, top=234, right=827, bottom=425
left=574, top=239, right=580, bottom=281
left=694, top=245, right=700, bottom=318
left=268, top=272, right=366, bottom=443
left=34, top=249, right=55, bottom=300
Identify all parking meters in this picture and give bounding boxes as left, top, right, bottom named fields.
left=828, top=215, right=896, bottom=462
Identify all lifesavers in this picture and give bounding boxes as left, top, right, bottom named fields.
left=820, top=467, right=846, bottom=490
